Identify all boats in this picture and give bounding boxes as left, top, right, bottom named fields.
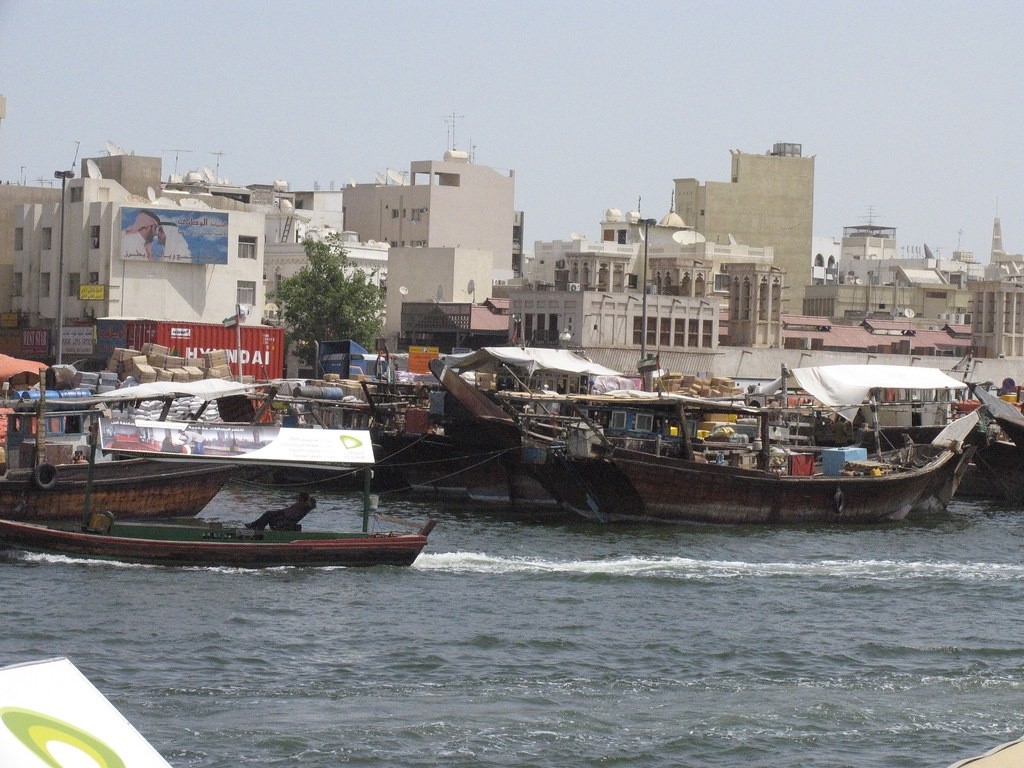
left=0, top=417, right=438, bottom=567
left=1, top=345, right=1024, bottom=527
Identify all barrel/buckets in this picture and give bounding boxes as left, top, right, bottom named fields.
left=319, top=386, right=343, bottom=400
left=293, top=386, right=322, bottom=399
left=753, top=438, right=762, bottom=450
left=11, top=389, right=92, bottom=412
left=369, top=494, right=379, bottom=511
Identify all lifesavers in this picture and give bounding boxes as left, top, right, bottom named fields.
left=34, top=463, right=59, bottom=490
left=834, top=491, right=844, bottom=513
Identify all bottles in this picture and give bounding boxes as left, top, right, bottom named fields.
left=717, top=451, right=724, bottom=466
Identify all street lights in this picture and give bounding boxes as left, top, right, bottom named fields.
left=637, top=219, right=657, bottom=360
left=54, top=170, right=75, bottom=364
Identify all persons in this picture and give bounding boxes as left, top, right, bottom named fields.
left=121, top=210, right=192, bottom=263
left=245, top=492, right=309, bottom=530
left=160, top=429, right=206, bottom=455
